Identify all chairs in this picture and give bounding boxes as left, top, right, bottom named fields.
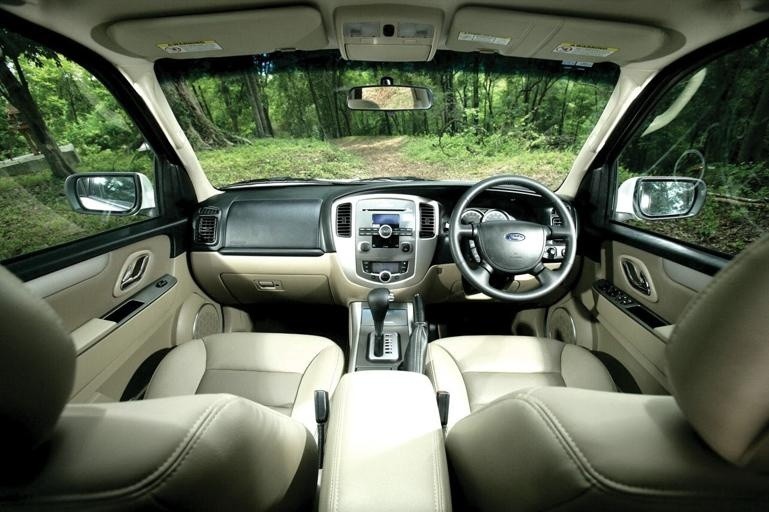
left=419, top=226, right=767, bottom=510
left=1, top=259, right=348, bottom=511
